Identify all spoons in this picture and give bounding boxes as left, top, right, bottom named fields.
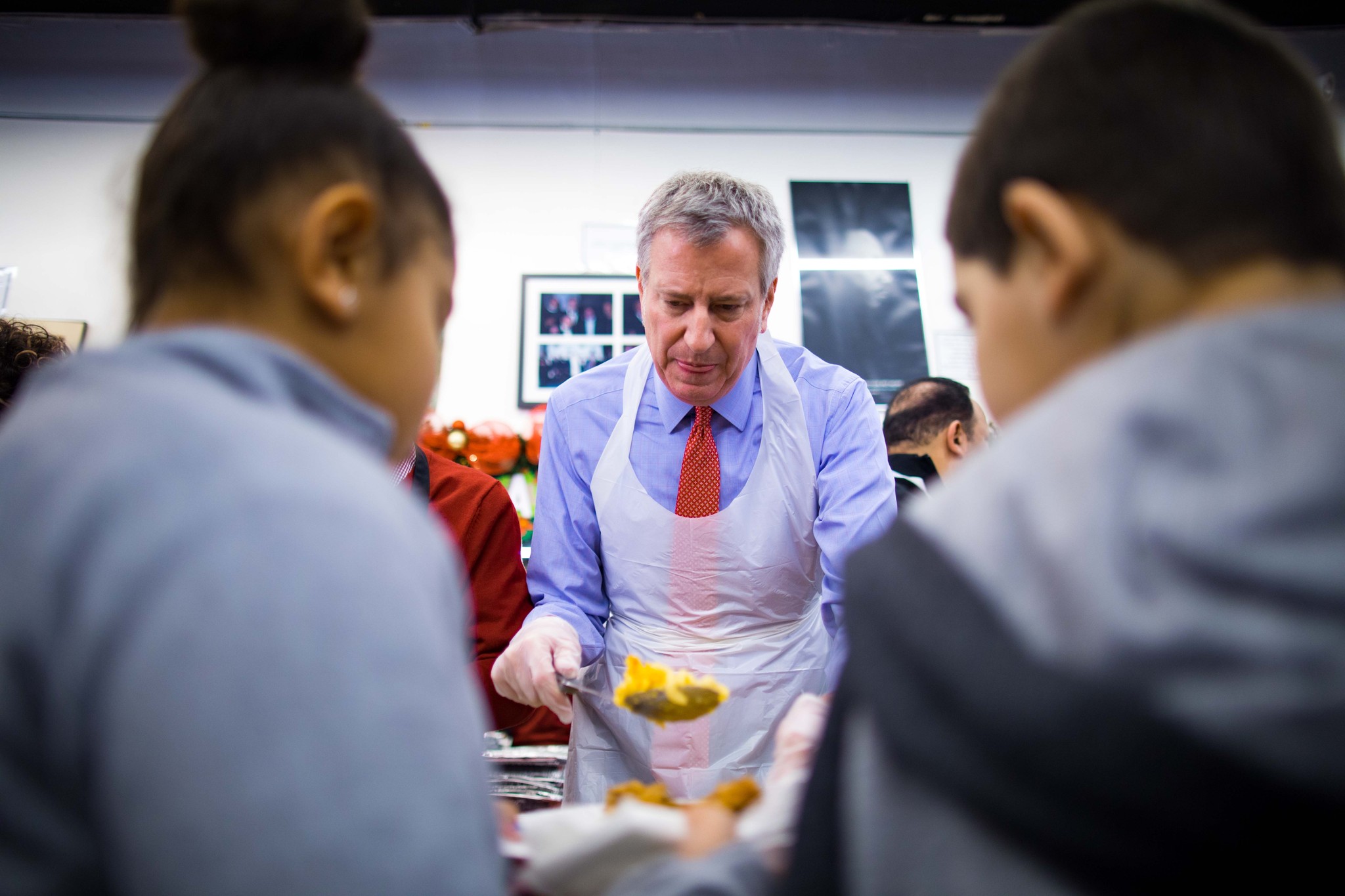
left=554, top=671, right=720, bottom=726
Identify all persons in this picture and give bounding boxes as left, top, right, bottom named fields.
left=3, top=61, right=513, bottom=895
left=536, top=292, right=648, bottom=390
left=490, top=169, right=888, bottom=894
left=387, top=438, right=580, bottom=747
left=783, top=0, right=1342, bottom=893
left=880, top=376, right=987, bottom=515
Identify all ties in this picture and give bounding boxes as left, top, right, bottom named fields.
left=645, top=407, right=722, bottom=785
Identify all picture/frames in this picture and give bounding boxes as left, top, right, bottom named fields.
left=516, top=273, right=647, bottom=409
